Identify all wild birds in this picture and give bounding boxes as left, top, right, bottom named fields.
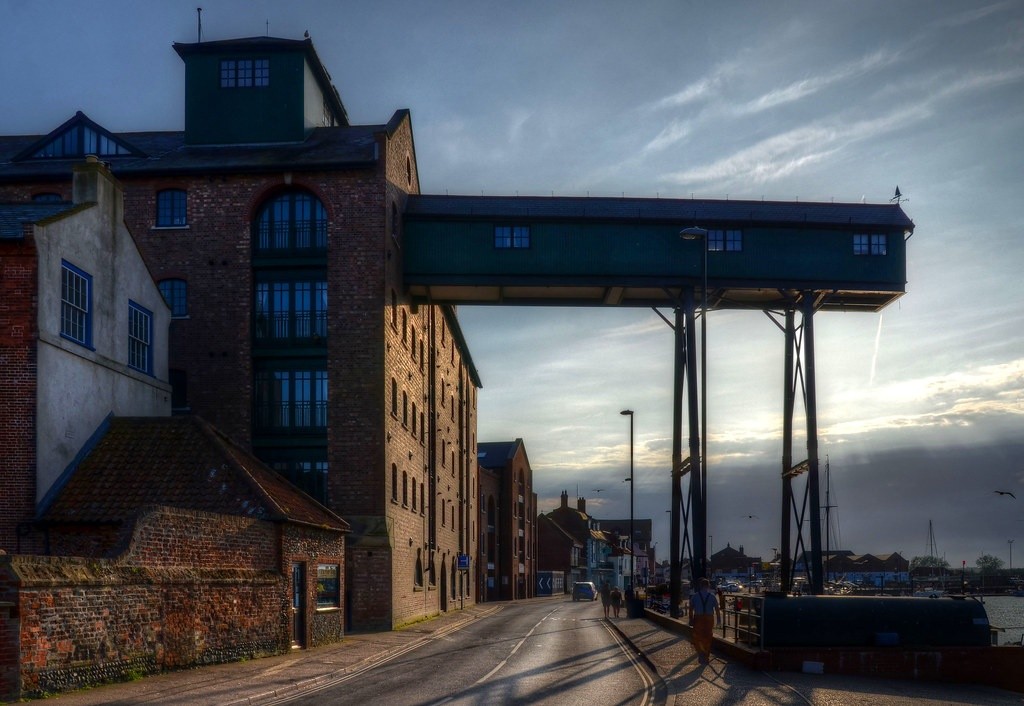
left=992, top=490, right=1018, bottom=499
left=740, top=514, right=759, bottom=521
left=592, top=489, right=605, bottom=492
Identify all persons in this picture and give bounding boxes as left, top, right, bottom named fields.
left=601, top=583, right=622, bottom=619
left=624, top=585, right=635, bottom=619
left=689, top=579, right=721, bottom=665
left=725, top=598, right=743, bottom=611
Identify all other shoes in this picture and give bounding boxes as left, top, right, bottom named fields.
left=698, top=651, right=710, bottom=665
left=615, top=616, right=619, bottom=618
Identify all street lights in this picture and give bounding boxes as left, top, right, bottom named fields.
left=621, top=408, right=634, bottom=589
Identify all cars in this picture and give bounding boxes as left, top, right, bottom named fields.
left=722, top=584, right=739, bottom=592
left=573, top=581, right=598, bottom=602
left=747, top=581, right=759, bottom=587
